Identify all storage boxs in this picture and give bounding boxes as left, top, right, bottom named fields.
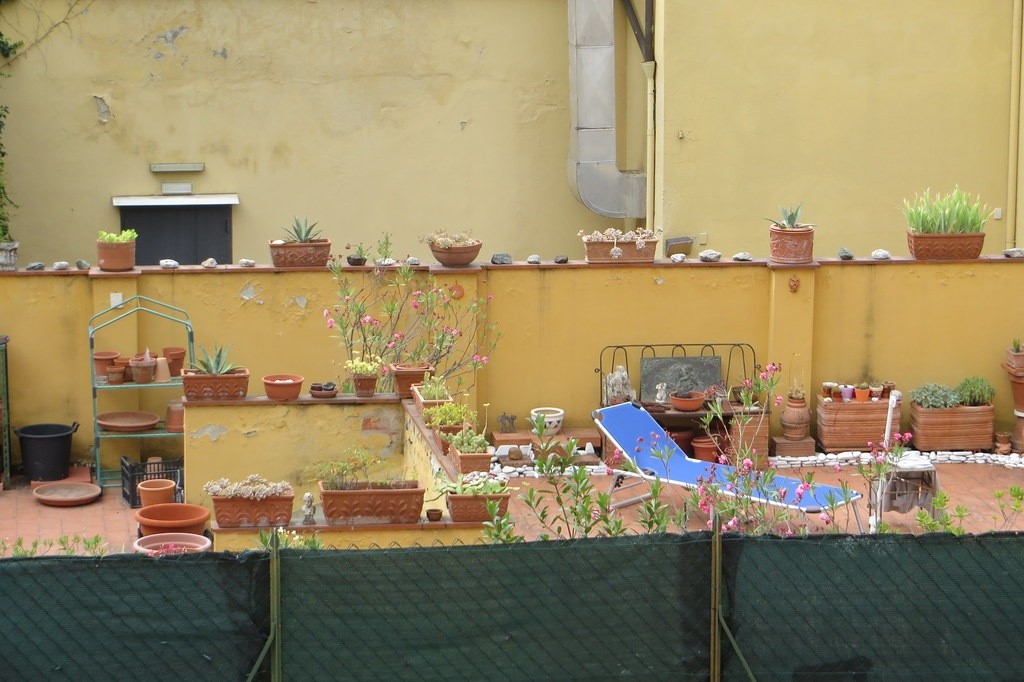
left=120, top=456, right=184, bottom=508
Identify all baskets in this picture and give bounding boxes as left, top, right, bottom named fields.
left=120, top=456, right=184, bottom=508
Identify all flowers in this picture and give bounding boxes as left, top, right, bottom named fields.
left=577, top=225, right=663, bottom=259
left=204, top=474, right=291, bottom=502
left=482, top=362, right=914, bottom=539
left=321, top=231, right=501, bottom=375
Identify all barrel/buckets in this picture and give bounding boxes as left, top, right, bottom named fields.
left=11, top=422, right=79, bottom=482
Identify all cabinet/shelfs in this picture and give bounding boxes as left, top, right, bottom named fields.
left=89, top=295, right=195, bottom=488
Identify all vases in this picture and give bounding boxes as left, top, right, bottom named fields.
left=92, top=347, right=186, bottom=432
left=692, top=436, right=715, bottom=445
left=582, top=234, right=658, bottom=263
left=388, top=362, right=436, bottom=398
left=670, top=430, right=694, bottom=455
left=262, top=375, right=305, bottom=399
left=132, top=479, right=212, bottom=556
left=691, top=442, right=720, bottom=463
left=212, top=486, right=295, bottom=528
left=779, top=399, right=812, bottom=441
left=351, top=375, right=378, bottom=397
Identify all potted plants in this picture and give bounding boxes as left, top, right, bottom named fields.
left=821, top=378, right=996, bottom=451
left=763, top=202, right=818, bottom=263
left=181, top=339, right=250, bottom=401
left=347, top=243, right=372, bottom=265
left=668, top=364, right=705, bottom=411
left=97, top=228, right=139, bottom=272
left=410, top=370, right=492, bottom=474
left=530, top=407, right=563, bottom=435
left=269, top=215, right=332, bottom=267
left=304, top=444, right=425, bottom=524
left=898, top=184, right=994, bottom=260
left=1006, top=336, right=1024, bottom=368
left=417, top=226, right=482, bottom=267
left=426, top=468, right=511, bottom=522
left=0, top=214, right=18, bottom=271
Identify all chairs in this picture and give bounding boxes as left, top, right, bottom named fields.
left=591, top=398, right=865, bottom=534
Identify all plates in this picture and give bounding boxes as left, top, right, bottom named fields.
left=97, top=411, right=160, bottom=431
left=33, top=481, right=102, bottom=506
